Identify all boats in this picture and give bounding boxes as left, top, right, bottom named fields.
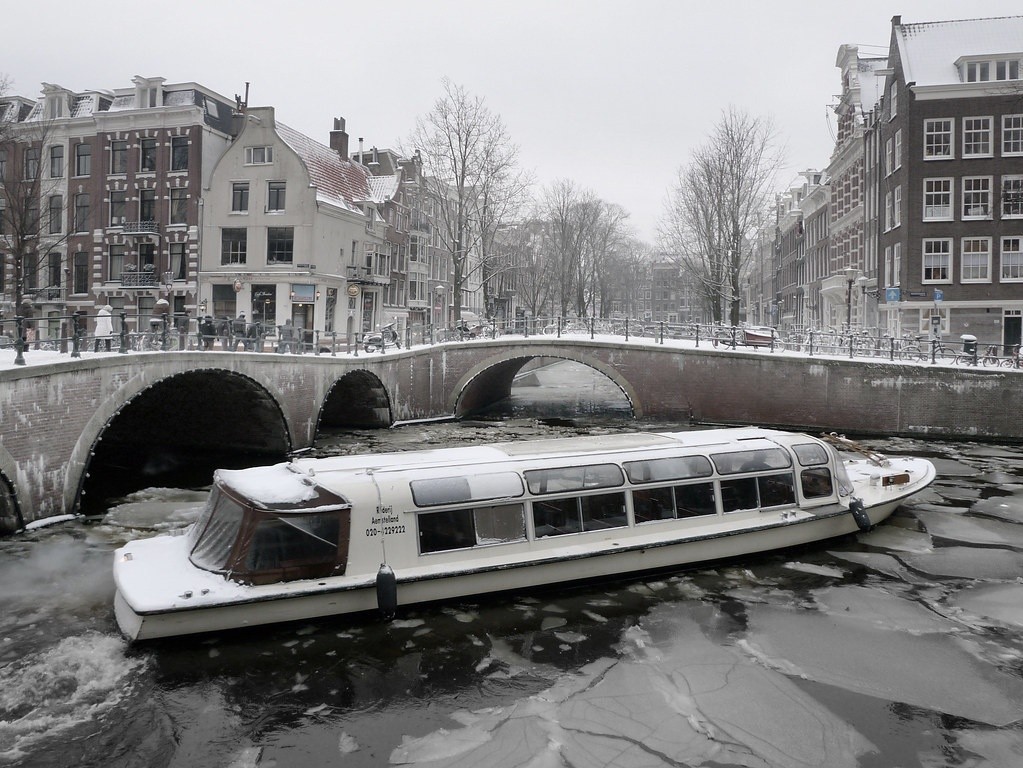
left=111, top=425, right=938, bottom=643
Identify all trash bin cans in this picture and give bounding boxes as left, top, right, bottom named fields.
left=960, top=335, right=976, bottom=355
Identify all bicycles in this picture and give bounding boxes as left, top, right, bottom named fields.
left=543, top=317, right=922, bottom=361
left=38, top=329, right=179, bottom=352
left=900, top=336, right=956, bottom=366
left=956, top=347, right=999, bottom=368
left=452, top=312, right=493, bottom=343
left=1000, top=349, right=1022, bottom=368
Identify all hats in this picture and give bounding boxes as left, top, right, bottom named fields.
left=239, top=311, right=246, bottom=316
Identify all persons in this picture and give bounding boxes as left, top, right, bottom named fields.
left=144, top=296, right=170, bottom=349
left=278, top=319, right=296, bottom=354
left=738, top=451, right=774, bottom=508
left=17, top=299, right=35, bottom=348
left=94, top=305, right=113, bottom=352
left=169, top=310, right=192, bottom=351
left=202, top=313, right=264, bottom=351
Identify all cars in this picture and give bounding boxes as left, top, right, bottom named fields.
left=479, top=326, right=500, bottom=338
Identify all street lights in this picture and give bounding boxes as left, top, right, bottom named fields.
left=263, top=299, right=270, bottom=331
left=844, top=266, right=858, bottom=333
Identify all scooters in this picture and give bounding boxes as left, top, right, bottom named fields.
left=363, top=316, right=403, bottom=353
